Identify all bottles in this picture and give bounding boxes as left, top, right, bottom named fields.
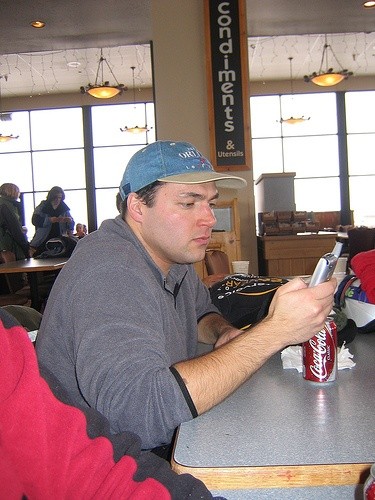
left=363, top=464, right=375, bottom=500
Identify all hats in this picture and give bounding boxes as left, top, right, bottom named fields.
left=116, top=139, right=247, bottom=209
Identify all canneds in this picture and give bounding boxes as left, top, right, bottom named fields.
left=303, top=317, right=336, bottom=384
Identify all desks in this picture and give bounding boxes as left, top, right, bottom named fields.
left=0, top=257, right=70, bottom=310
left=171, top=276, right=375, bottom=489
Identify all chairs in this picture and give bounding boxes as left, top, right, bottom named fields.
left=205, top=249, right=230, bottom=274
left=0, top=249, right=55, bottom=305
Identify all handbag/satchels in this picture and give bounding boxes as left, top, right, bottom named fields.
left=211, top=269, right=290, bottom=331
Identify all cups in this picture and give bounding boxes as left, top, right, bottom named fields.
left=334, top=257, right=348, bottom=274
left=232, top=261, right=249, bottom=275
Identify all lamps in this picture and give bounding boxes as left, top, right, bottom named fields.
left=276, top=56, right=310, bottom=124
left=304, top=34, right=353, bottom=87
left=119, top=66, right=153, bottom=133
left=0, top=74, right=20, bottom=142
left=86, top=48, right=128, bottom=99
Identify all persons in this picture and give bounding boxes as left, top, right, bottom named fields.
left=0, top=306, right=228, bottom=500
left=0, top=183, right=31, bottom=294
left=36, top=141, right=337, bottom=451
left=30, top=187, right=73, bottom=249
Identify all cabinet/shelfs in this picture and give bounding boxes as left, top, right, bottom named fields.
left=257, top=234, right=337, bottom=277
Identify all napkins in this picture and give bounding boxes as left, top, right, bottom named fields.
left=281, top=339, right=356, bottom=373
